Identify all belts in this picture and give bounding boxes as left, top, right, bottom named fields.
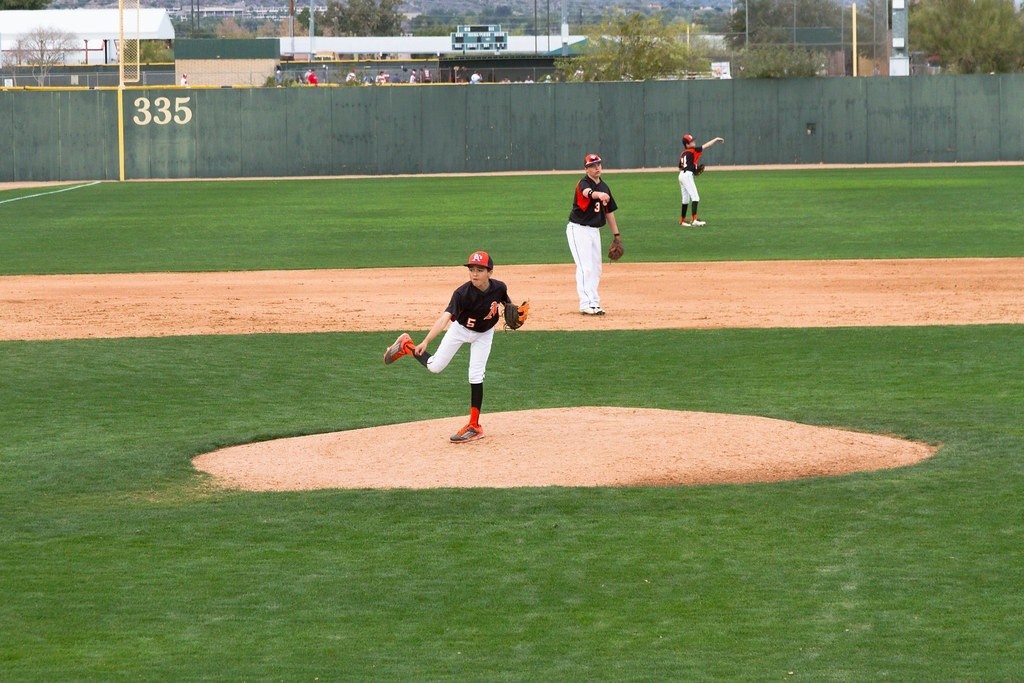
left=580, top=223, right=598, bottom=227
left=684, top=170, right=687, bottom=173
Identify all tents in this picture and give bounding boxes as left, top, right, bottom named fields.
left=544, top=35, right=629, bottom=57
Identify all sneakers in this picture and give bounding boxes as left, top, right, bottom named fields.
left=579, top=306, right=606, bottom=315
left=691, top=220, right=707, bottom=226
left=679, top=221, right=691, bottom=227
left=384, top=333, right=414, bottom=364
left=450, top=424, right=484, bottom=443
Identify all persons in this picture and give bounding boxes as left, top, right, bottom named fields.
left=384, top=251, right=529, bottom=443
left=816, top=62, right=916, bottom=76
left=450, top=66, right=586, bottom=83
left=347, top=70, right=357, bottom=83
left=364, top=65, right=433, bottom=83
left=678, top=133, right=724, bottom=227
left=566, top=154, right=623, bottom=316
left=275, top=65, right=282, bottom=84
left=181, top=74, right=188, bottom=86
left=297, top=69, right=318, bottom=84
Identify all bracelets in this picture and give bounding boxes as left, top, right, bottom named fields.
left=614, top=233, right=620, bottom=236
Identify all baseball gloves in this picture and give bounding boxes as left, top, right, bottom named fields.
left=607, top=238, right=625, bottom=260
left=504, top=301, right=529, bottom=330
left=695, top=164, right=705, bottom=176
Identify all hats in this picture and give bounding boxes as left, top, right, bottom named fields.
left=682, top=135, right=695, bottom=143
left=464, top=251, right=494, bottom=270
left=584, top=154, right=601, bottom=166
